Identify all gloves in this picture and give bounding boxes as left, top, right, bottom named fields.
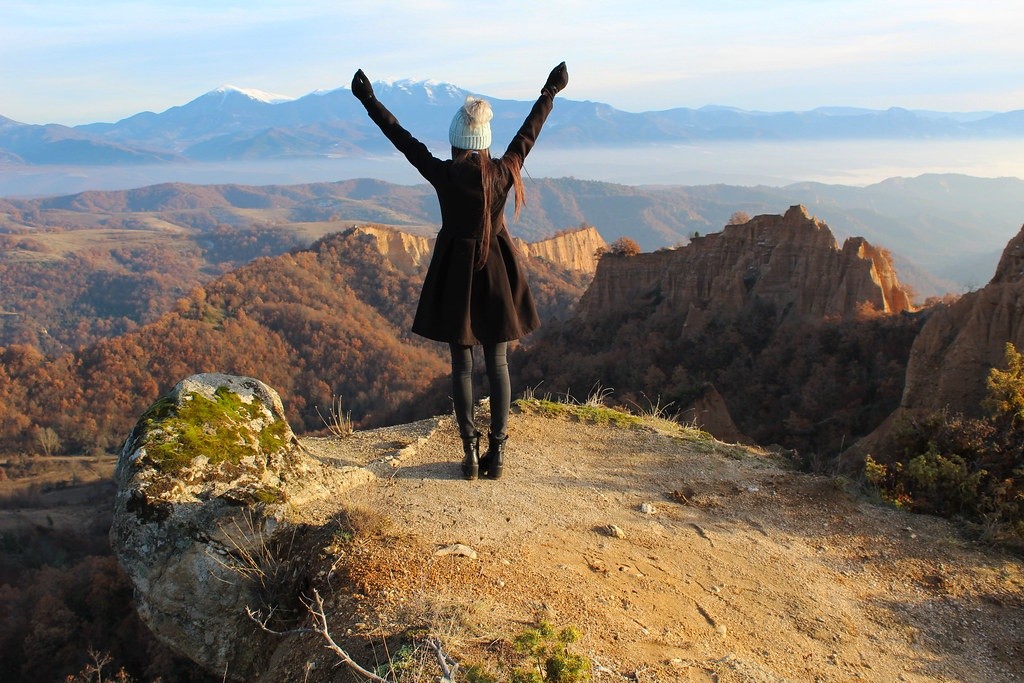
left=540, top=61, right=568, bottom=102
left=351, top=69, right=378, bottom=111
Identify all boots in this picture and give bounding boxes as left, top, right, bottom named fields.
left=479, top=428, right=509, bottom=477
left=460, top=431, right=483, bottom=480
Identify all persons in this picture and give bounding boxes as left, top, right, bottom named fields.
left=352, top=61, right=568, bottom=480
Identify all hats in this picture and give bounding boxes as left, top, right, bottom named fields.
left=448, top=96, right=493, bottom=150
